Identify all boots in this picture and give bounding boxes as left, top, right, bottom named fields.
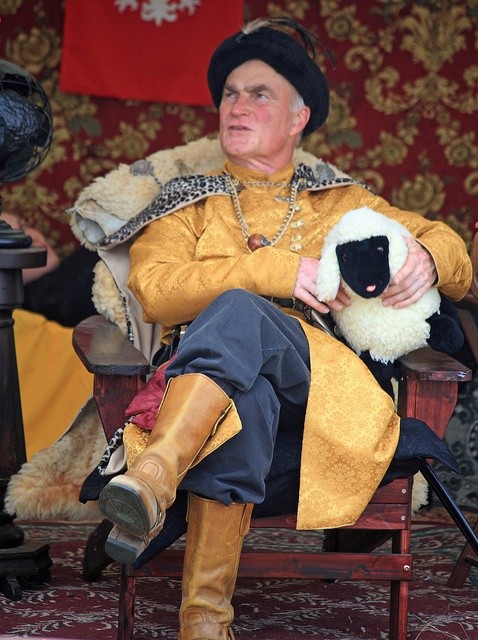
left=100, top=374, right=232, bottom=564
left=178, top=493, right=255, bottom=640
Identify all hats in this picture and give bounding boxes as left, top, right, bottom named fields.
left=207, top=16, right=329, bottom=137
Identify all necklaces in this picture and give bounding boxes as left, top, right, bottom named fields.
left=220, top=172, right=302, bottom=251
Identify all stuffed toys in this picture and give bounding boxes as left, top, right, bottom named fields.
left=315, top=205, right=441, bottom=364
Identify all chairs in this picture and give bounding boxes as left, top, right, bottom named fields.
left=69, top=317, right=473, bottom=634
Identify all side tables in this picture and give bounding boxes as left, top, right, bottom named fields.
left=0, top=250, right=53, bottom=603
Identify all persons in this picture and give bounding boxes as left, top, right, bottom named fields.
left=78, top=20, right=474, bottom=636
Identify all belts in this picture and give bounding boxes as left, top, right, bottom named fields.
left=260, top=294, right=310, bottom=315
